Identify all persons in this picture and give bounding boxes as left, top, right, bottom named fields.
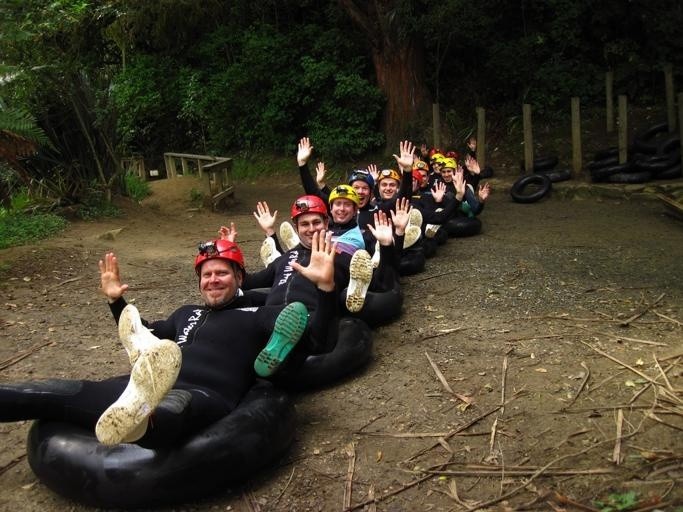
left=254, top=183, right=414, bottom=313
left=279, top=137, right=416, bottom=278
left=1, top=232, right=339, bottom=447
left=117, top=195, right=395, bottom=377
left=315, top=136, right=491, bottom=248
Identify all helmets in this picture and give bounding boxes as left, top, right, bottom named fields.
left=290, top=196, right=329, bottom=224
left=412, top=148, right=457, bottom=185
left=348, top=168, right=374, bottom=189
left=194, top=239, right=246, bottom=277
left=377, top=169, right=400, bottom=185
left=328, top=184, right=360, bottom=207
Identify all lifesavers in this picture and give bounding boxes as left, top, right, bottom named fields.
left=27, top=375, right=296, bottom=510
left=584, top=123, right=683, bottom=184
left=536, top=165, right=571, bottom=182
left=521, top=154, right=557, bottom=171
left=400, top=211, right=482, bottom=275
left=283, top=315, right=372, bottom=392
left=511, top=173, right=551, bottom=201
left=461, top=165, right=493, bottom=178
left=337, top=263, right=404, bottom=325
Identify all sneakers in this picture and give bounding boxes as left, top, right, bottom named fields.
left=403, top=209, right=423, bottom=250
left=461, top=184, right=474, bottom=218
left=280, top=221, right=300, bottom=250
left=95, top=338, right=183, bottom=448
left=260, top=237, right=282, bottom=269
left=254, top=302, right=308, bottom=377
left=118, top=304, right=160, bottom=366
left=425, top=207, right=444, bottom=238
left=344, top=249, right=373, bottom=313
left=370, top=235, right=396, bottom=269
